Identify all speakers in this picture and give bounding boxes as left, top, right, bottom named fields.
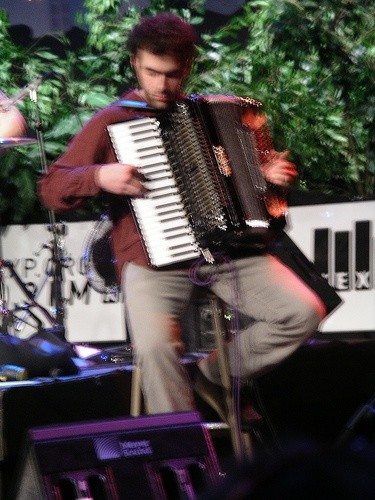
left=16, top=410, right=223, bottom=500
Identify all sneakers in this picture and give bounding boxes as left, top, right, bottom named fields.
left=192, top=366, right=261, bottom=427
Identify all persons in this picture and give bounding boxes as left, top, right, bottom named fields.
left=38, top=10, right=327, bottom=431
left=0, top=89, right=28, bottom=141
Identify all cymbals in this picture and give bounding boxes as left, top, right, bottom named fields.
left=0, top=136, right=38, bottom=150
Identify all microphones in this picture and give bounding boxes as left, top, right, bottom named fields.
left=0, top=71, right=57, bottom=113
